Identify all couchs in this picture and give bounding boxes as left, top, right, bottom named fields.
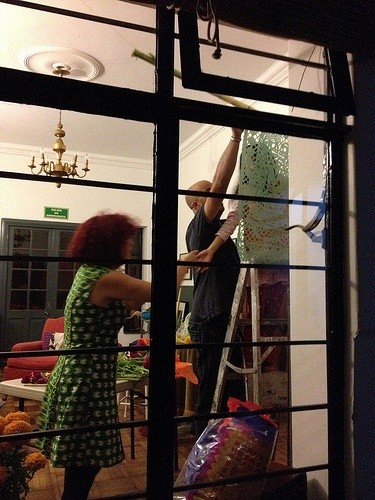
left=2, top=316, right=64, bottom=401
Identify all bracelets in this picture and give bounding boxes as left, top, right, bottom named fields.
left=230, top=136, right=242, bottom=142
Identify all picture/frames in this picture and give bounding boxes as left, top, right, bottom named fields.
left=179, top=253, right=192, bottom=280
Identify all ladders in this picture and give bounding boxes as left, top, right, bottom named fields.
left=208, top=259, right=290, bottom=419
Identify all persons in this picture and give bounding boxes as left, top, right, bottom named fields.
left=36, top=213, right=199, bottom=500
left=185, top=127, right=247, bottom=442
left=196, top=144, right=290, bottom=372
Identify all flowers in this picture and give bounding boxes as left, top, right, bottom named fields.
left=0, top=410, right=49, bottom=500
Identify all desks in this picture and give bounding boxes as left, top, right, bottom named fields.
left=0, top=378, right=135, bottom=460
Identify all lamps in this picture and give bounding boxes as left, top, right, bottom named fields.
left=28, top=69, right=90, bottom=188
